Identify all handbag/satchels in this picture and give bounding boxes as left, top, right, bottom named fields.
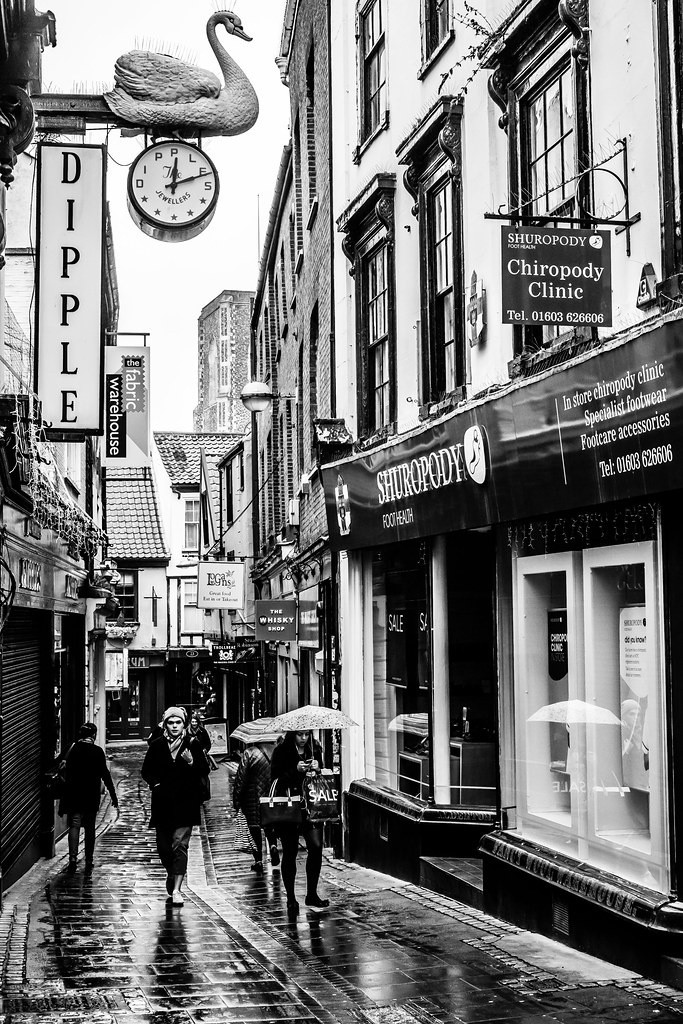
left=232, top=808, right=258, bottom=854
left=56, top=742, right=75, bottom=786
left=303, top=772, right=340, bottom=823
left=189, top=736, right=211, bottom=801
left=259, top=779, right=304, bottom=836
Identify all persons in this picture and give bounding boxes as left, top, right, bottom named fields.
left=621, top=699, right=649, bottom=790
left=142, top=706, right=212, bottom=905
left=232, top=742, right=280, bottom=872
left=269, top=729, right=330, bottom=912
left=57, top=722, right=118, bottom=872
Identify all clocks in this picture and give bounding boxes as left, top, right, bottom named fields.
left=131, top=143, right=215, bottom=225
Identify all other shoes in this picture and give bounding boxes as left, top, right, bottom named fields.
left=287, top=899, right=299, bottom=908
left=85, top=862, right=94, bottom=868
left=69, top=856, right=76, bottom=861
left=270, top=847, right=280, bottom=866
left=172, top=890, right=184, bottom=904
left=305, top=896, right=330, bottom=907
left=166, top=874, right=175, bottom=896
left=251, top=862, right=263, bottom=869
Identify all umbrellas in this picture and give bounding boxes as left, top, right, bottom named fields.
left=526, top=698, right=621, bottom=726
left=388, top=712, right=429, bottom=737
left=229, top=718, right=287, bottom=745
left=261, top=704, right=360, bottom=760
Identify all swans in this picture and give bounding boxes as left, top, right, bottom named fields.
left=102, top=9, right=260, bottom=136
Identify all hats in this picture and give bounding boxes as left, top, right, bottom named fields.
left=163, top=706, right=186, bottom=723
